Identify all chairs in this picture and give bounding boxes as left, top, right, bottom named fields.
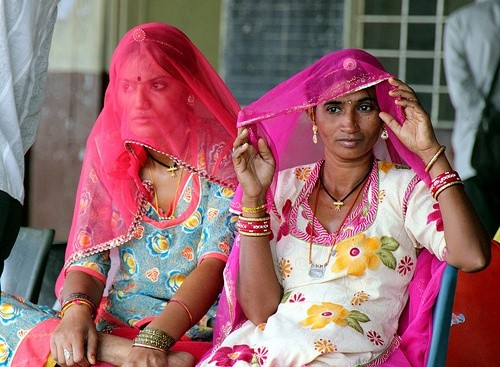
left=371, top=247, right=458, bottom=367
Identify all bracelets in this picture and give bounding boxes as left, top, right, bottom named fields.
left=242, top=205, right=265, bottom=214
left=166, top=300, right=193, bottom=328
left=59, top=293, right=97, bottom=319
left=238, top=213, right=271, bottom=237
left=425, top=144, right=446, bottom=173
left=430, top=170, right=463, bottom=200
left=132, top=328, right=176, bottom=354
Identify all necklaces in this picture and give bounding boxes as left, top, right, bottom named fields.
left=144, top=146, right=179, bottom=178
left=145, top=152, right=186, bottom=219
left=308, top=160, right=374, bottom=279
left=318, top=160, right=372, bottom=212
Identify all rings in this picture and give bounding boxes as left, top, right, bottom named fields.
left=232, top=147, right=235, bottom=152
left=63, top=349, right=73, bottom=360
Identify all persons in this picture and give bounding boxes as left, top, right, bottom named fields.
left=0, top=0, right=58, bottom=277
left=444, top=0, right=500, bottom=240
left=7, top=25, right=250, bottom=367
left=183, top=48, right=492, bottom=367
